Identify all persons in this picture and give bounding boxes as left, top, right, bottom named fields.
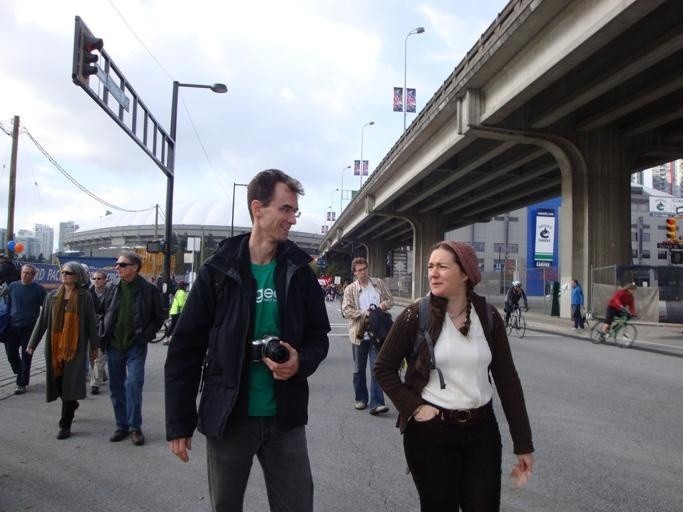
left=341, top=257, right=395, bottom=416
left=0, top=252, right=190, bottom=397
left=570, top=278, right=584, bottom=330
left=599, top=283, right=636, bottom=339
left=371, top=240, right=534, bottom=512
left=25, top=261, right=101, bottom=438
left=94, top=253, right=163, bottom=445
left=503, top=280, right=527, bottom=327
left=162, top=168, right=330, bottom=512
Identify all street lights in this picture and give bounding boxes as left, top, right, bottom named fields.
left=161, top=80, right=229, bottom=296
left=360, top=119, right=376, bottom=196
left=401, top=23, right=427, bottom=138
left=339, top=164, right=352, bottom=215
left=323, top=205, right=334, bottom=236
left=329, top=187, right=341, bottom=229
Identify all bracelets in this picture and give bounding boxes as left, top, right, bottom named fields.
left=412, top=403, right=423, bottom=417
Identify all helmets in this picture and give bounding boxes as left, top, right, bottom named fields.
left=512, top=280, right=522, bottom=288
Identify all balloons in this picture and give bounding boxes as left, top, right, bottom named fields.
left=15, top=243, right=24, bottom=254
left=6, top=241, right=15, bottom=252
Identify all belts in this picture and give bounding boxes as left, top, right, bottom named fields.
left=421, top=398, right=494, bottom=423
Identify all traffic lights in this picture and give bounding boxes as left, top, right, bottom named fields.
left=664, top=217, right=677, bottom=239
left=79, top=31, right=102, bottom=82
left=145, top=240, right=161, bottom=256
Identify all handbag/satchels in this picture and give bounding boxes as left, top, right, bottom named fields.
left=1, top=285, right=12, bottom=342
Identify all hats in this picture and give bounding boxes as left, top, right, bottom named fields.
left=438, top=240, right=482, bottom=286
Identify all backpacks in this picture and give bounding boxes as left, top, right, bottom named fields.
left=364, top=302, right=395, bottom=352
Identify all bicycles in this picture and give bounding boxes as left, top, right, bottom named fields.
left=500, top=304, right=528, bottom=339
left=144, top=313, right=171, bottom=349
left=588, top=307, right=643, bottom=349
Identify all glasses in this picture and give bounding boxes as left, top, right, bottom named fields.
left=115, top=261, right=135, bottom=267
left=92, top=277, right=104, bottom=280
left=61, top=271, right=76, bottom=275
left=264, top=202, right=302, bottom=218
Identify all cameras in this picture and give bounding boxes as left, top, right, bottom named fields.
left=251, top=335, right=289, bottom=364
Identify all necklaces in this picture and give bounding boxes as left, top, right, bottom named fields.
left=447, top=298, right=468, bottom=320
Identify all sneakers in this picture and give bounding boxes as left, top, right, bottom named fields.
left=354, top=400, right=366, bottom=409
left=369, top=405, right=389, bottom=415
left=14, top=384, right=26, bottom=395
left=90, top=386, right=99, bottom=395
left=129, top=426, right=145, bottom=446
left=56, top=427, right=70, bottom=439
left=109, top=426, right=130, bottom=442
